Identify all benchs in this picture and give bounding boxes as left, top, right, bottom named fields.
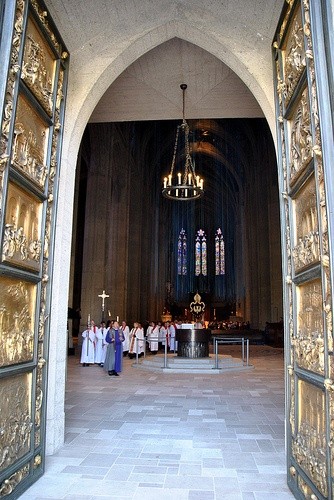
left=210, top=329, right=241, bottom=344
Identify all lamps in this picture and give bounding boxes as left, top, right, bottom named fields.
left=161, top=84, right=204, bottom=201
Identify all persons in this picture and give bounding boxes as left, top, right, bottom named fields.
left=280, top=317, right=283, bottom=323
left=93, top=321, right=108, bottom=366
left=106, top=320, right=111, bottom=330
left=129, top=321, right=144, bottom=359
left=209, top=321, right=249, bottom=329
left=119, top=320, right=129, bottom=356
left=264, top=322, right=269, bottom=333
left=105, top=322, right=125, bottom=376
left=157, top=320, right=178, bottom=353
left=112, top=320, right=115, bottom=324
left=80, top=325, right=95, bottom=367
left=91, top=319, right=98, bottom=332
left=178, top=319, right=206, bottom=329
left=146, top=321, right=159, bottom=354
left=143, top=320, right=149, bottom=336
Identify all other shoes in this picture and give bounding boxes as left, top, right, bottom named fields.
left=83, top=363, right=89, bottom=367
left=99, top=363, right=104, bottom=367
left=108, top=371, right=113, bottom=375
left=114, top=372, right=119, bottom=376
